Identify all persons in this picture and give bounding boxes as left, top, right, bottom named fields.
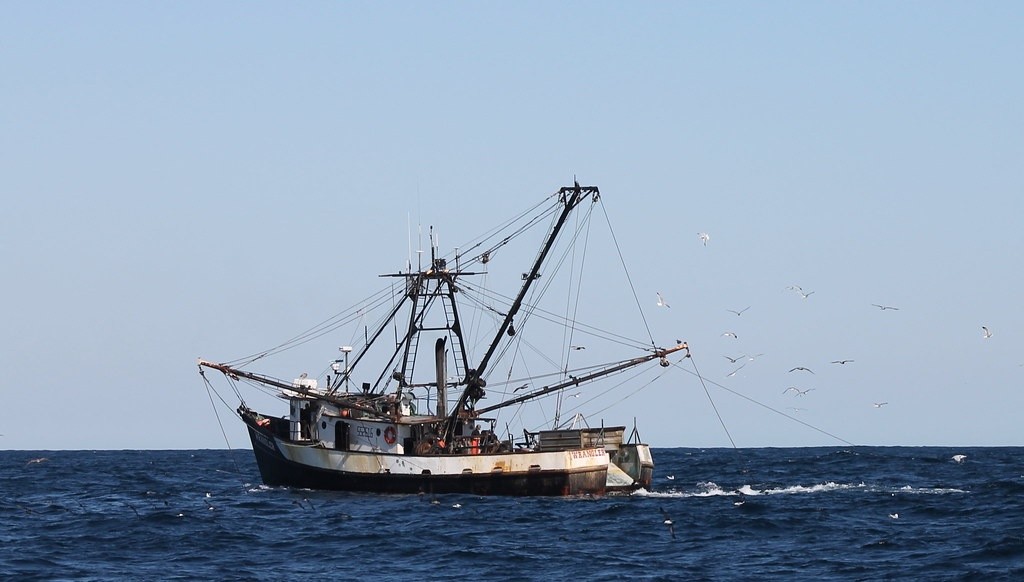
left=470, top=424, right=481, bottom=442
left=303, top=403, right=311, bottom=439
left=437, top=426, right=446, bottom=444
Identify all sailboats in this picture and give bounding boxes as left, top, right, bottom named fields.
left=195, top=173, right=689, bottom=496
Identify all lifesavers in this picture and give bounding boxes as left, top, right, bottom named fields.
left=384, top=426, right=397, bottom=444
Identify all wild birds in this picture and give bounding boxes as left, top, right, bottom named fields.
left=872, top=304, right=899, bottom=311
left=831, top=360, right=853, bottom=365
left=720, top=332, right=737, bottom=339
left=659, top=506, right=676, bottom=539
left=872, top=402, right=887, bottom=407
left=656, top=292, right=671, bottom=308
left=565, top=392, right=583, bottom=399
left=16, top=488, right=464, bottom=522
left=23, top=458, right=50, bottom=467
left=697, top=232, right=710, bottom=246
left=786, top=285, right=815, bottom=299
left=726, top=306, right=751, bottom=316
left=723, top=352, right=765, bottom=377
left=569, top=346, right=586, bottom=351
left=788, top=367, right=813, bottom=374
left=513, top=383, right=530, bottom=394
left=982, top=326, right=994, bottom=339
left=786, top=406, right=808, bottom=413
left=953, top=455, right=968, bottom=464
left=783, top=386, right=816, bottom=397
